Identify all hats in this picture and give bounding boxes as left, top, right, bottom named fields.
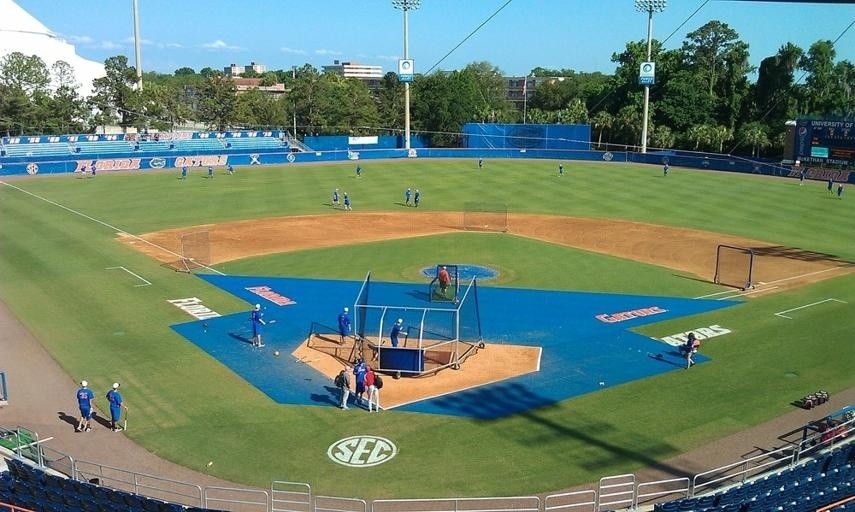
left=79, top=380, right=88, bottom=386
left=112, top=382, right=121, bottom=389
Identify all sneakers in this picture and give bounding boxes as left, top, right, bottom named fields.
left=76, top=426, right=84, bottom=432
left=113, top=426, right=123, bottom=432
left=85, top=426, right=92, bottom=432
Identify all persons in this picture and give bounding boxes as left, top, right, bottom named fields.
left=478, top=157, right=483, bottom=168
left=356, top=163, right=362, bottom=176
left=207, top=164, right=213, bottom=180
left=663, top=163, right=670, bottom=175
left=390, top=317, right=408, bottom=348
left=405, top=188, right=411, bottom=205
left=104, top=382, right=127, bottom=433
left=440, top=266, right=451, bottom=298
left=331, top=188, right=341, bottom=208
left=685, top=332, right=696, bottom=370
left=251, top=303, right=266, bottom=349
left=799, top=171, right=805, bottom=184
left=334, top=365, right=352, bottom=411
left=343, top=191, right=352, bottom=211
left=181, top=164, right=187, bottom=180
left=559, top=162, right=563, bottom=176
left=352, top=358, right=366, bottom=403
left=337, top=306, right=351, bottom=345
left=828, top=178, right=832, bottom=197
left=837, top=183, right=843, bottom=198
left=80, top=165, right=86, bottom=178
left=413, top=189, right=421, bottom=206
left=75, top=380, right=96, bottom=433
left=362, top=365, right=379, bottom=413
left=226, top=162, right=234, bottom=175
left=91, top=165, right=96, bottom=177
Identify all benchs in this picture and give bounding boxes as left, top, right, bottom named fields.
left=1, top=136, right=289, bottom=158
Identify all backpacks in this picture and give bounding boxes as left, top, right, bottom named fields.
left=374, top=376, right=383, bottom=388
left=334, top=374, right=345, bottom=388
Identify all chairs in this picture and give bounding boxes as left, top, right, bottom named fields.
left=0, top=459, right=228, bottom=512
left=653, top=439, right=855, bottom=512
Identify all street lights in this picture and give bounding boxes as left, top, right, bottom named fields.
left=512, top=72, right=537, bottom=125
left=634, top=0, right=668, bottom=155
left=391, top=0, right=422, bottom=149
